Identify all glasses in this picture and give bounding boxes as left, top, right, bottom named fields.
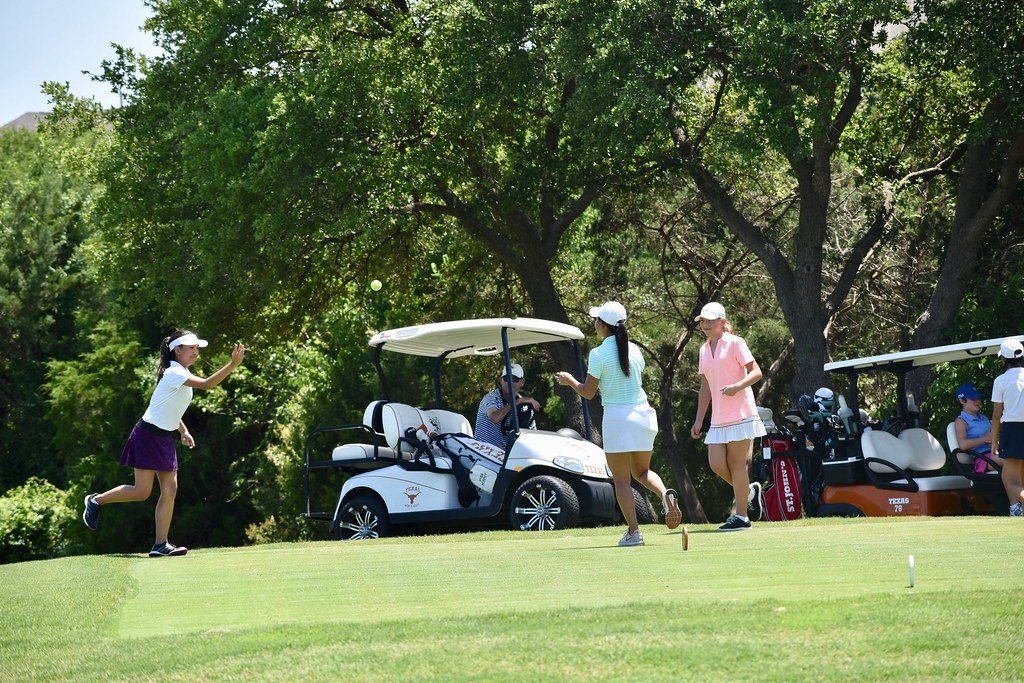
left=503, top=375, right=522, bottom=382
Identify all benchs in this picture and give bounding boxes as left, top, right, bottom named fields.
left=860, top=422, right=1005, bottom=494
left=307, top=401, right=472, bottom=471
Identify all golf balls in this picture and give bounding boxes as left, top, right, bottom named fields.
left=371, top=279, right=382, bottom=292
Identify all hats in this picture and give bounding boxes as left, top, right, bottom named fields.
left=998, top=339, right=1024, bottom=358
left=956, top=384, right=986, bottom=400
left=501, top=364, right=524, bottom=378
left=694, top=302, right=725, bottom=321
left=169, top=334, right=208, bottom=352
left=589, top=301, right=626, bottom=326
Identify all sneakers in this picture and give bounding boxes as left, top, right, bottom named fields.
left=661, top=489, right=682, bottom=530
left=1010, top=504, right=1024, bottom=516
left=619, top=529, right=643, bottom=546
left=748, top=482, right=762, bottom=520
left=718, top=516, right=752, bottom=531
left=149, top=541, right=187, bottom=557
left=83, top=493, right=103, bottom=531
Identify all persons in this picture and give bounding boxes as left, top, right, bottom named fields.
left=954, top=384, right=1024, bottom=515
left=82, top=329, right=245, bottom=556
left=992, top=340, right=1024, bottom=505
left=554, top=301, right=682, bottom=546
left=476, top=364, right=541, bottom=449
left=691, top=302, right=768, bottom=530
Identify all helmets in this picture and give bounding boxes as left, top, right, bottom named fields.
left=814, top=388, right=834, bottom=411
